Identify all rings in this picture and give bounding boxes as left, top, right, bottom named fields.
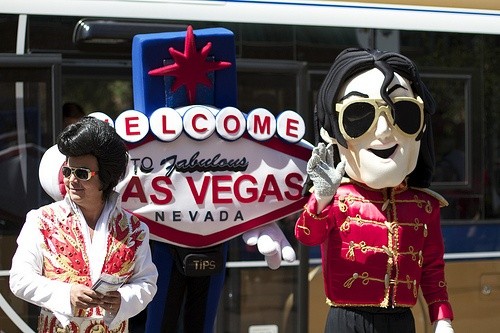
left=109, top=305, right=113, bottom=309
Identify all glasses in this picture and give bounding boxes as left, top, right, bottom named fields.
left=61, top=166, right=100, bottom=182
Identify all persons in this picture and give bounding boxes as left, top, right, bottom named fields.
left=9, top=115, right=158, bottom=333
left=295, top=46, right=455, bottom=333
left=62, top=101, right=86, bottom=128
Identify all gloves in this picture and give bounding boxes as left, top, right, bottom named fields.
left=307, top=143, right=346, bottom=200
left=433, top=321, right=455, bottom=333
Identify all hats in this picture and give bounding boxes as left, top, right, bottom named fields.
left=62, top=102, right=88, bottom=118
